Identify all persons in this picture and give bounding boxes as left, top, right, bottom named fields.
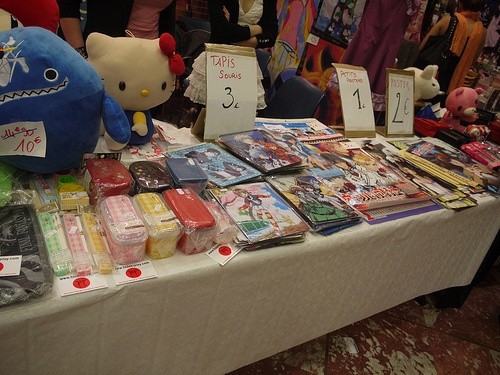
left=411, top=0, right=487, bottom=109
left=483, top=0, right=500, bottom=54
left=56, top=0, right=173, bottom=60
left=191, top=0, right=278, bottom=125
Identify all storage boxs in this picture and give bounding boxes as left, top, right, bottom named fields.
left=83, top=156, right=216, bottom=264
left=459, top=140, right=500, bottom=170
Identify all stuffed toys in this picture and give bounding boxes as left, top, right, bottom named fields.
left=438, top=86, right=490, bottom=144
left=0, top=26, right=130, bottom=174
left=85, top=32, right=186, bottom=146
left=0, top=0, right=59, bottom=35
left=402, top=65, right=440, bottom=101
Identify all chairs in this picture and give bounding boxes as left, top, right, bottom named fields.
left=259, top=75, right=325, bottom=119
left=172, top=16, right=272, bottom=127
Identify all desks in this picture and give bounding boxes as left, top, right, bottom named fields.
left=0, top=117, right=500, bottom=375
